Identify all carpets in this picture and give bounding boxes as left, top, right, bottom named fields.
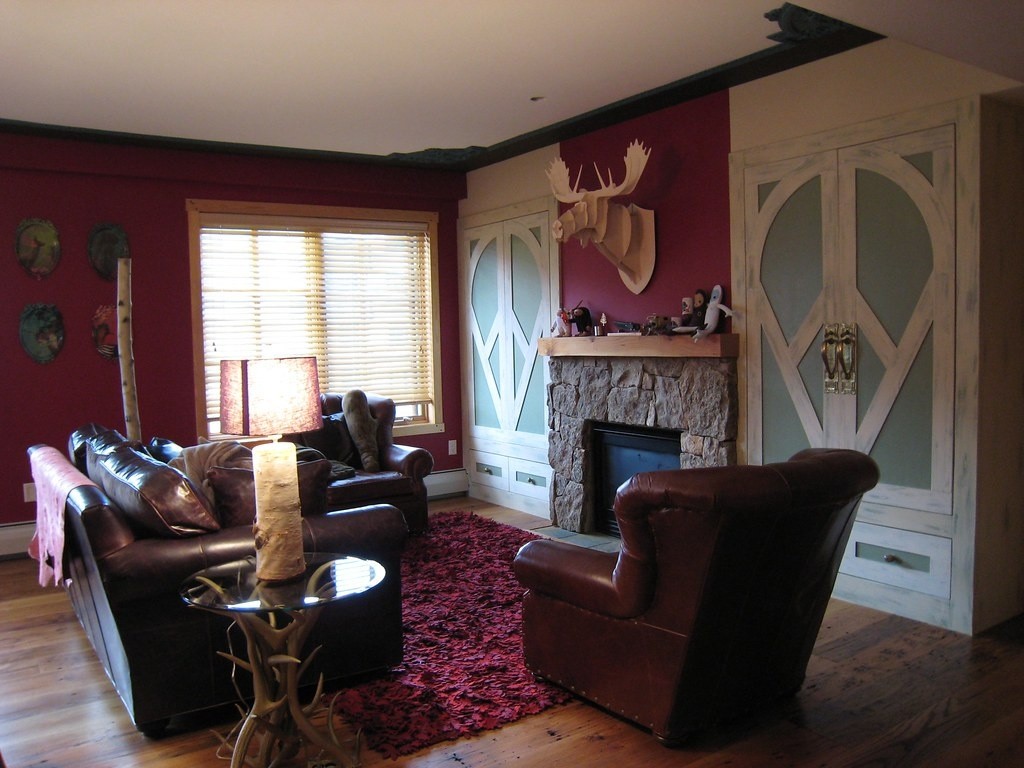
left=318, top=510, right=577, bottom=760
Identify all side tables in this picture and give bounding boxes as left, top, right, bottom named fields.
left=178, top=552, right=387, bottom=768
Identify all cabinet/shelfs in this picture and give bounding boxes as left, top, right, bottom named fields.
left=455, top=195, right=562, bottom=521
left=729, top=94, right=1024, bottom=636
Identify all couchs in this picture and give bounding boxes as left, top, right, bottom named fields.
left=27, top=421, right=402, bottom=741
left=513, top=448, right=881, bottom=748
left=286, top=389, right=435, bottom=534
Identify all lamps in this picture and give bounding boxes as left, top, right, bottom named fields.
left=219, top=356, right=325, bottom=579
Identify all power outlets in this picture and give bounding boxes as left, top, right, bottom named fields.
left=449, top=440, right=457, bottom=456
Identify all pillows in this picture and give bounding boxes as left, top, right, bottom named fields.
left=206, top=459, right=331, bottom=526
